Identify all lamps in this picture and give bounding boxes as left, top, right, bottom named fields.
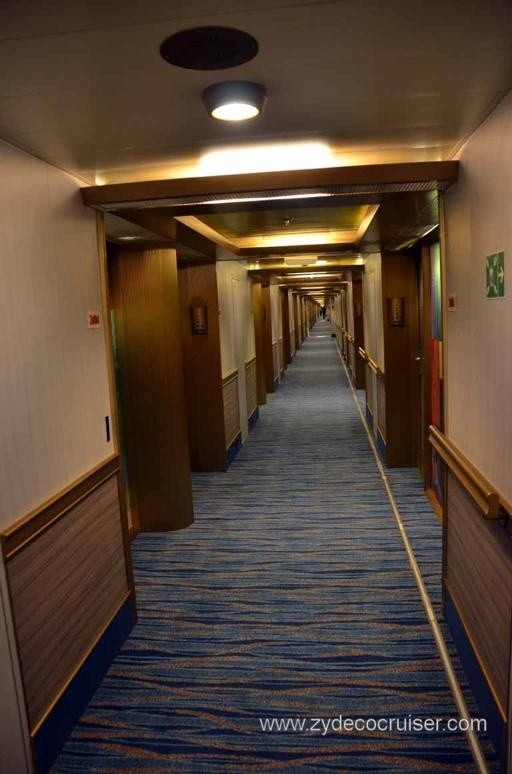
left=202, top=79, right=267, bottom=122
